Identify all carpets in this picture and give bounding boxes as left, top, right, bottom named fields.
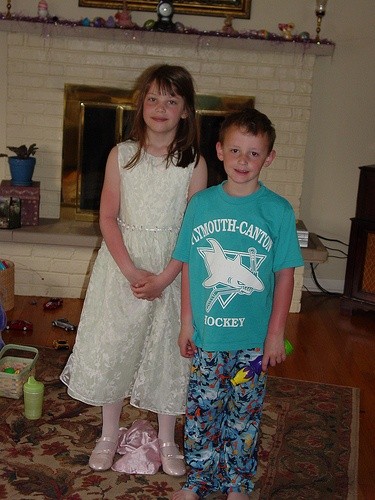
left=0, top=343, right=361, bottom=500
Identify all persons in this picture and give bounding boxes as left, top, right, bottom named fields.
left=169, top=107, right=305, bottom=500
left=59, top=65, right=207, bottom=476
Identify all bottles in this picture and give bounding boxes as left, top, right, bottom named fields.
left=231, top=339, right=295, bottom=386
left=23, top=375, right=44, bottom=420
left=37, top=0, right=49, bottom=20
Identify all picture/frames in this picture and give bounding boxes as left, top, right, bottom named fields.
left=78, top=0, right=251, bottom=20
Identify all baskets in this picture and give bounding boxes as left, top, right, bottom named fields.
left=0, top=343, right=40, bottom=399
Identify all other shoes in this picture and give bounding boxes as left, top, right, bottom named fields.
left=88, top=437, right=118, bottom=472
left=159, top=442, right=186, bottom=476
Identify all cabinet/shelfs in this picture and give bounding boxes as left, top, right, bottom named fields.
left=338, top=164, right=375, bottom=316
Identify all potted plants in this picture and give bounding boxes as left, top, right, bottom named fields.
left=0, top=143, right=40, bottom=187
